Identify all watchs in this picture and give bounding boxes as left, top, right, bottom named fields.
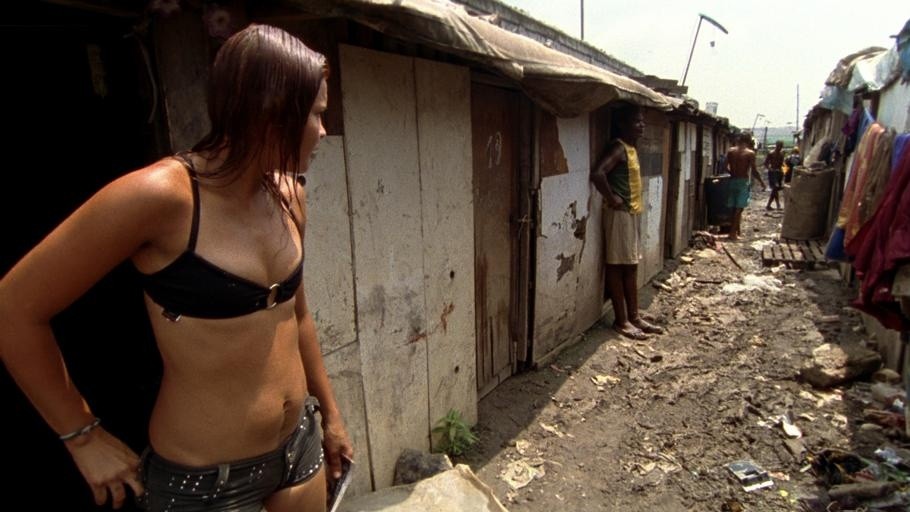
left=722, top=130, right=767, bottom=243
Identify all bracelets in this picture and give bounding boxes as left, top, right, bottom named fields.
left=56, top=414, right=101, bottom=443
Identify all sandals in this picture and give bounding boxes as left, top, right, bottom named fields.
left=612, top=319, right=663, bottom=340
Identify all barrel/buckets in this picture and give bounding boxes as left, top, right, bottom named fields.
left=705, top=173, right=731, bottom=224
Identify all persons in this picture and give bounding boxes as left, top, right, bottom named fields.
left=589, top=105, right=664, bottom=341
left=763, top=139, right=786, bottom=211
left=0, top=21, right=357, bottom=512
left=783, top=145, right=802, bottom=184
